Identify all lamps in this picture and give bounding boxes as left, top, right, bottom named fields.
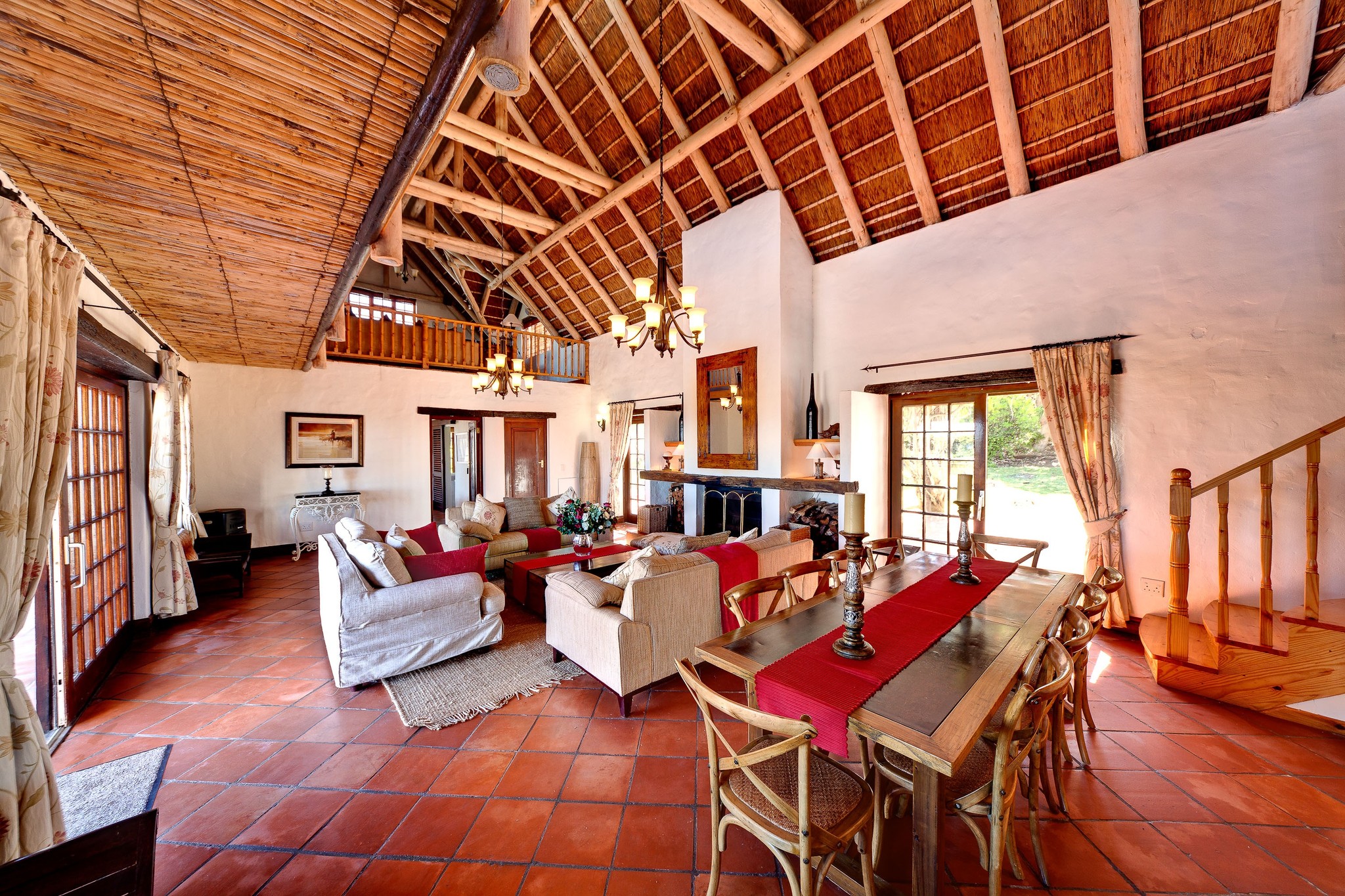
left=392, top=256, right=419, bottom=285
left=805, top=442, right=833, bottom=479
left=719, top=364, right=745, bottom=415
left=608, top=0, right=705, bottom=358
left=661, top=452, right=674, bottom=471
left=835, top=454, right=840, bottom=479
left=500, top=314, right=523, bottom=346
left=472, top=155, right=534, bottom=399
left=674, top=443, right=685, bottom=471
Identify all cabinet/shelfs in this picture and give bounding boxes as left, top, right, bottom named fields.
left=199, top=508, right=247, bottom=535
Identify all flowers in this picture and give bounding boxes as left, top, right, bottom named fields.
left=551, top=499, right=618, bottom=535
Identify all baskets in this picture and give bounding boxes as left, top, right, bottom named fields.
left=637, top=504, right=668, bottom=534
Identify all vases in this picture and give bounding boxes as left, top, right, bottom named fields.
left=572, top=533, right=593, bottom=554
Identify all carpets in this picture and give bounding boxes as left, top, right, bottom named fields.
left=383, top=577, right=587, bottom=728
left=53, top=744, right=174, bottom=844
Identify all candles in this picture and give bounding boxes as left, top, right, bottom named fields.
left=957, top=474, right=972, bottom=501
left=324, top=466, right=332, bottom=477
left=844, top=491, right=865, bottom=533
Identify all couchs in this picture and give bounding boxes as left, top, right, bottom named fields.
left=438, top=494, right=614, bottom=575
left=544, top=524, right=816, bottom=719
left=318, top=532, right=506, bottom=692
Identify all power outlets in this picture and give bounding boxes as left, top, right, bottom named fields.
left=300, top=521, right=314, bottom=532
left=1139, top=576, right=1165, bottom=597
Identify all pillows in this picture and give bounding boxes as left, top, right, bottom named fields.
left=346, top=538, right=412, bottom=588
left=676, top=529, right=731, bottom=555
left=730, top=526, right=759, bottom=543
left=470, top=494, right=507, bottom=536
left=547, top=486, right=580, bottom=518
left=545, top=568, right=624, bottom=609
left=178, top=528, right=198, bottom=561
left=502, top=496, right=546, bottom=532
left=335, top=517, right=384, bottom=545
left=448, top=519, right=493, bottom=542
left=376, top=521, right=444, bottom=554
left=383, top=524, right=427, bottom=557
left=600, top=545, right=664, bottom=584
left=405, top=542, right=490, bottom=582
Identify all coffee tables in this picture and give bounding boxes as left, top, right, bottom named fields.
left=503, top=540, right=644, bottom=618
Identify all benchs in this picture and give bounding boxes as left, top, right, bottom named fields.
left=187, top=533, right=255, bottom=599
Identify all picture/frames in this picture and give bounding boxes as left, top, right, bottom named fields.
left=285, top=411, right=364, bottom=468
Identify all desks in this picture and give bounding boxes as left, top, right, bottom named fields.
left=290, top=490, right=366, bottom=561
left=696, top=551, right=1085, bottom=896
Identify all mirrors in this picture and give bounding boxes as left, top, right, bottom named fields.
left=696, top=347, right=759, bottom=469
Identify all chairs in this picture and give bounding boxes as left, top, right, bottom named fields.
left=674, top=530, right=1124, bottom=896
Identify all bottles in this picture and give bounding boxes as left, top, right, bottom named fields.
left=679, top=392, right=683, bottom=442
left=806, top=373, right=818, bottom=440
